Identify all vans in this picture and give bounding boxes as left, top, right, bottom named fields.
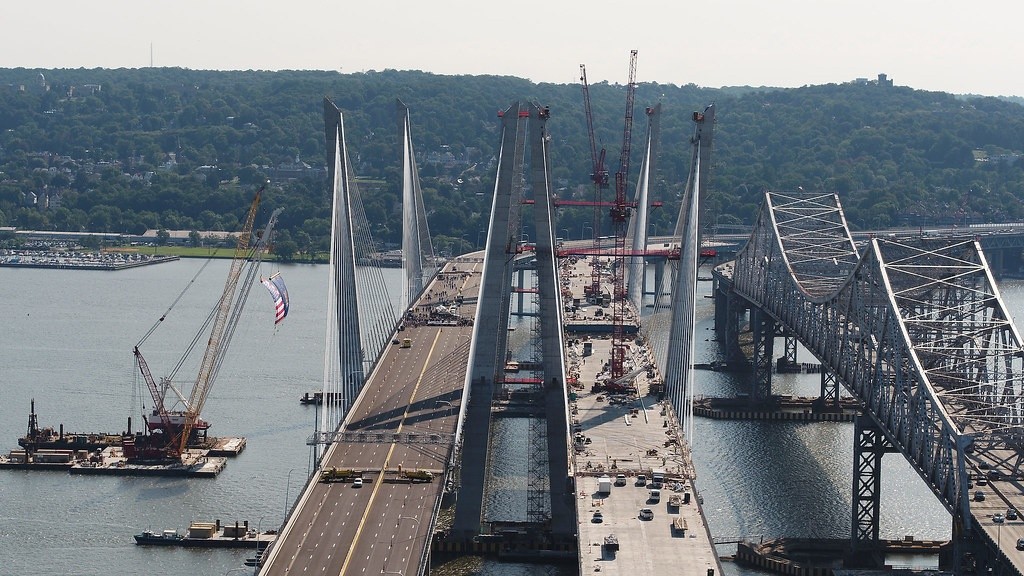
left=354, top=477, right=363, bottom=488
left=648, top=489, right=660, bottom=503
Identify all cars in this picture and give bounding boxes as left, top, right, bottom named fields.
left=593, top=513, right=604, bottom=523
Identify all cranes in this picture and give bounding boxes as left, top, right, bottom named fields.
left=128, top=180, right=287, bottom=450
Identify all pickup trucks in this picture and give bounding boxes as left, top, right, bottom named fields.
left=640, top=508, right=654, bottom=520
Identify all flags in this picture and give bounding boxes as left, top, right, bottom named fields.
left=260, top=273, right=290, bottom=325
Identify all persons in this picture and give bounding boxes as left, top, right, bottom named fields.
left=584, top=359, right=585, bottom=364
left=601, top=359, right=603, bottom=364
left=403, top=271, right=482, bottom=332
